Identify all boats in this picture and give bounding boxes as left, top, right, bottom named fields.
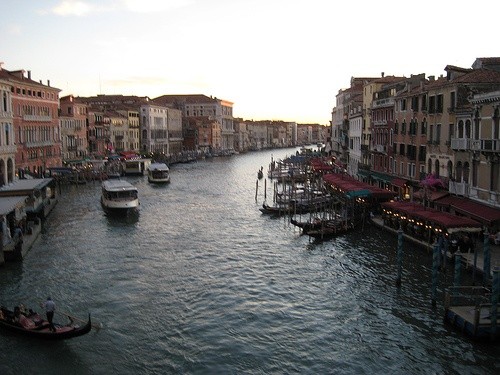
left=99, top=178, right=140, bottom=210
left=0, top=195, right=43, bottom=264
left=146, top=161, right=171, bottom=184
left=0, top=302, right=92, bottom=340
left=0, top=177, right=61, bottom=222
left=260, top=143, right=365, bottom=239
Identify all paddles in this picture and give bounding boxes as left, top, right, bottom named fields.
left=39, top=301, right=105, bottom=333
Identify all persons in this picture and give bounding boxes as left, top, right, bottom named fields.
left=40, top=295, right=58, bottom=331
